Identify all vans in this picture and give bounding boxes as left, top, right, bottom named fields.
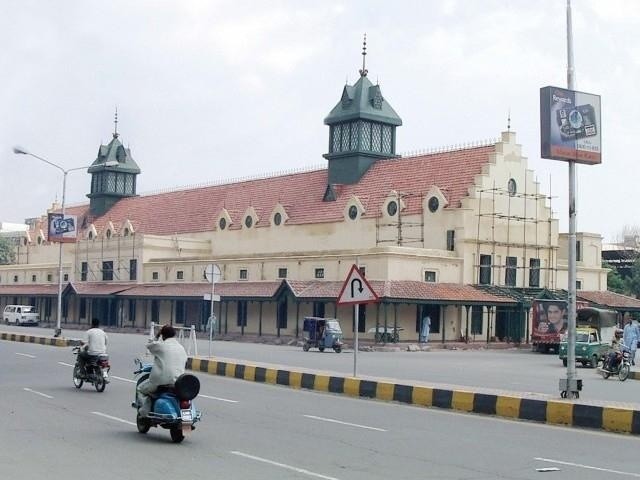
left=2, top=303, right=41, bottom=327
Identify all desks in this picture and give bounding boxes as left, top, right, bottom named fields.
left=368, top=325, right=404, bottom=343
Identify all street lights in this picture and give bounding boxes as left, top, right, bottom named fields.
left=12, top=143, right=120, bottom=338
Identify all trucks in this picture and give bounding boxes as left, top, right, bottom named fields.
left=529, top=297, right=623, bottom=369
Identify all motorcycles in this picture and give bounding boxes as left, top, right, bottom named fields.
left=130, top=357, right=202, bottom=443
left=72, top=339, right=111, bottom=393
left=596, top=340, right=633, bottom=381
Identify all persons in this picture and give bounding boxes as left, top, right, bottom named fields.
left=422, top=310, right=431, bottom=343
left=622, top=316, right=640, bottom=367
left=77, top=317, right=109, bottom=361
left=136, top=324, right=188, bottom=415
left=537, top=302, right=568, bottom=335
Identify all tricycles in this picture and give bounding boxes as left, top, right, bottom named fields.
left=302, top=316, right=344, bottom=354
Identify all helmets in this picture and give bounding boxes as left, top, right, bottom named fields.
left=614, top=329, right=624, bottom=338
